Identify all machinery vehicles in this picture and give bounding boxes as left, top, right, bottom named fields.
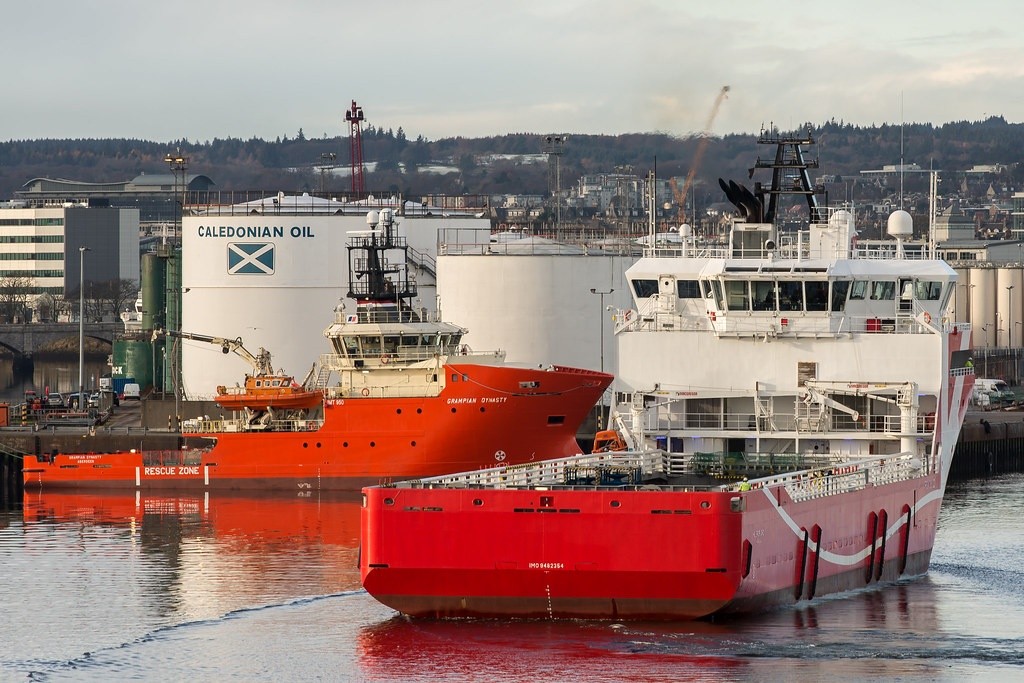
left=668, top=86, right=730, bottom=224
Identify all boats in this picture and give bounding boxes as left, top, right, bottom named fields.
left=22, top=202, right=616, bottom=493
left=213, top=347, right=330, bottom=411
left=357, top=134, right=978, bottom=622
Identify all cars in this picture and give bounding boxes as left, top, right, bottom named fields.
left=98, top=389, right=120, bottom=406
left=88, top=395, right=99, bottom=408
left=47, top=393, right=65, bottom=406
left=68, top=392, right=90, bottom=409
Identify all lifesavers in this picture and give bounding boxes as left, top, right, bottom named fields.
left=361, top=388, right=370, bottom=397
left=984, top=452, right=993, bottom=471
left=924, top=312, right=931, bottom=324
left=308, top=422, right=316, bottom=431
left=624, top=309, right=637, bottom=321
left=380, top=353, right=390, bottom=364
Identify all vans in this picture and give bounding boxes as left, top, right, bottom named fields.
left=124, top=383, right=140, bottom=400
left=973, top=379, right=1015, bottom=404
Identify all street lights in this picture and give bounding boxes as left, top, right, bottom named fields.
left=78, top=247, right=92, bottom=393
left=968, top=283, right=975, bottom=329
left=1006, top=286, right=1014, bottom=354
left=954, top=284, right=968, bottom=323
left=995, top=313, right=1004, bottom=348
left=981, top=324, right=992, bottom=378
left=1014, top=321, right=1023, bottom=385
left=590, top=289, right=615, bottom=429
